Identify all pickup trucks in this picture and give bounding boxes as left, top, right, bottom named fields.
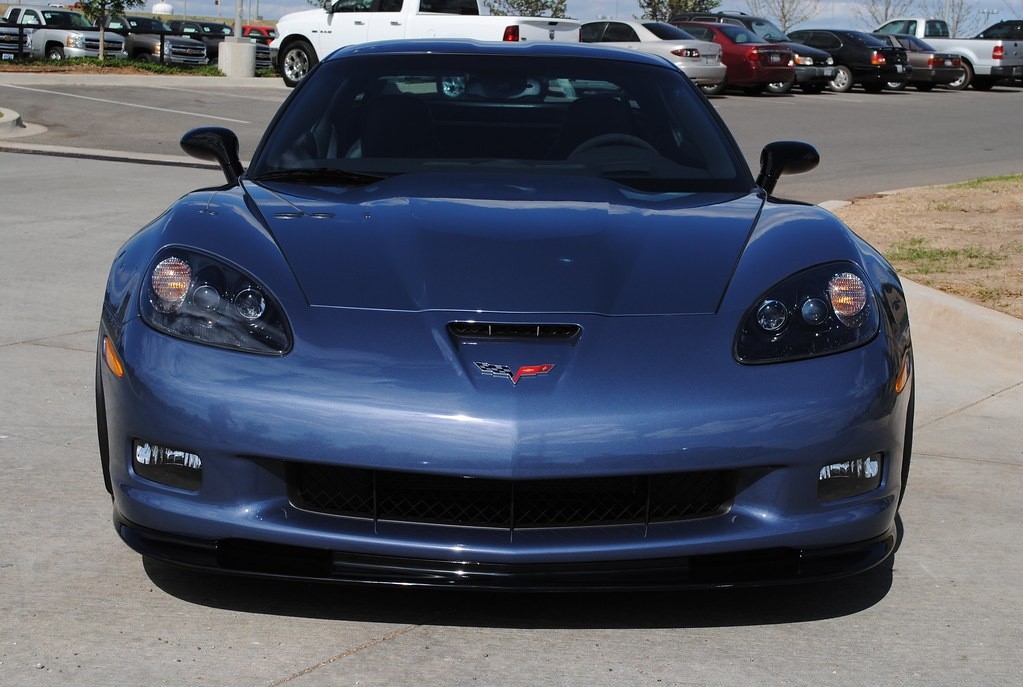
left=269, top=0, right=582, bottom=87
left=873, top=18, right=1023, bottom=92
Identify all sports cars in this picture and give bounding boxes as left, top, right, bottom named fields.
left=94, top=41, right=915, bottom=596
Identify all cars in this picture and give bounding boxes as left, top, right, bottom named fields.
left=579, top=12, right=960, bottom=97
left=0, top=5, right=277, bottom=70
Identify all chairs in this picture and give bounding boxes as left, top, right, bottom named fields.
left=928, top=23, right=940, bottom=36
left=362, top=94, right=445, bottom=157
left=546, top=97, right=634, bottom=162
